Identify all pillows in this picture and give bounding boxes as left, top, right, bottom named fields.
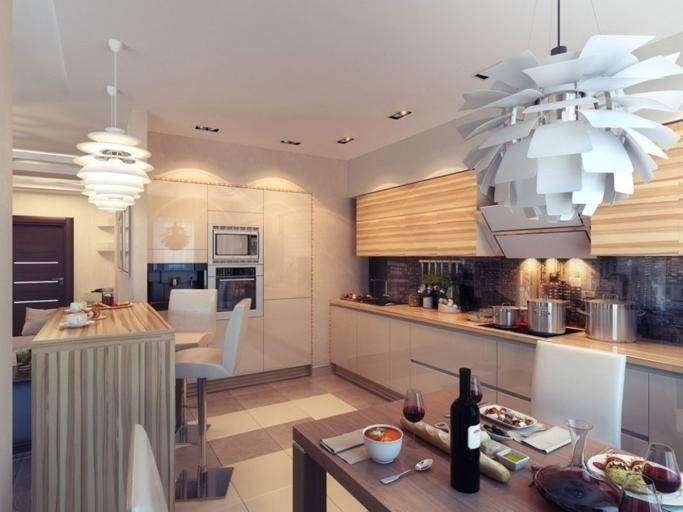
left=21, top=307, right=57, bottom=336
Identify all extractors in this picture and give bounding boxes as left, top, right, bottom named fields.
left=479, top=203, right=598, bottom=261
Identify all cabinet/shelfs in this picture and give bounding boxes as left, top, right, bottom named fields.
left=328, top=300, right=683, bottom=466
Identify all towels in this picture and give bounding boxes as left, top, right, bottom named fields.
left=319, top=424, right=373, bottom=465
left=514, top=425, right=580, bottom=454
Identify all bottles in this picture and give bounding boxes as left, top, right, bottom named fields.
left=449, top=366, right=482, bottom=494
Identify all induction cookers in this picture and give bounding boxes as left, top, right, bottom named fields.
left=476, top=324, right=580, bottom=340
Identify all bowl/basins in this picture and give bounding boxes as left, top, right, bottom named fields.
left=362, top=424, right=403, bottom=463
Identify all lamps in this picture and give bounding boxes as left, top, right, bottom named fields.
left=455, top=0, right=683, bottom=222
left=73, top=39, right=155, bottom=215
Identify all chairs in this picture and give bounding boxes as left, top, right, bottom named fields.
left=167, top=288, right=218, bottom=445
left=175, top=298, right=252, bottom=502
left=528, top=340, right=626, bottom=451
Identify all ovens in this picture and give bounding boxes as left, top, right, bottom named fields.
left=209, top=223, right=263, bottom=321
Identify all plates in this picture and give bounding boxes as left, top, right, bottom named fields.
left=479, top=404, right=538, bottom=428
left=586, top=454, right=683, bottom=507
left=93, top=315, right=109, bottom=320
left=58, top=321, right=95, bottom=326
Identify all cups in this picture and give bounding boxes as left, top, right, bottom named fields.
left=68, top=314, right=84, bottom=324
left=642, top=442, right=680, bottom=512
left=470, top=375, right=482, bottom=403
left=404, top=389, right=423, bottom=448
left=618, top=474, right=660, bottom=512
left=70, top=303, right=79, bottom=309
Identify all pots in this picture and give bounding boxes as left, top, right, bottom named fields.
left=578, top=301, right=646, bottom=343
left=493, top=303, right=521, bottom=325
left=525, top=298, right=568, bottom=334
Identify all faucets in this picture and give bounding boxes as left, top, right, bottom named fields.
left=370, top=278, right=388, bottom=297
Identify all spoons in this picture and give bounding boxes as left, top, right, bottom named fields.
left=380, top=458, right=432, bottom=485
